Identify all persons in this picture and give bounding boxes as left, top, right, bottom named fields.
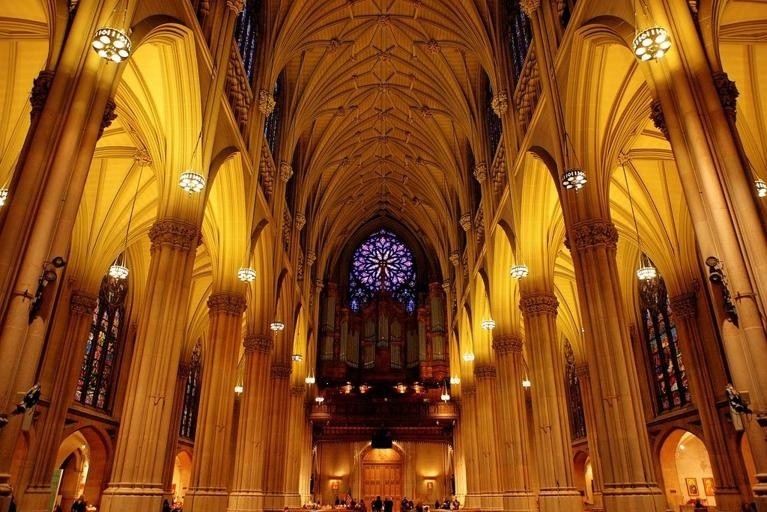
left=695, top=499, right=704, bottom=508
left=52, top=504, right=62, bottom=512
left=741, top=498, right=758, bottom=512
left=335, top=490, right=460, bottom=512
left=71, top=495, right=86, bottom=512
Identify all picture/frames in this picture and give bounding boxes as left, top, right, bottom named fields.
left=685, top=477, right=716, bottom=497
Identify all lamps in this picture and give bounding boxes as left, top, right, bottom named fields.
left=107, top=164, right=145, bottom=280
left=629, top=0, right=673, bottom=62
left=239, top=50, right=267, bottom=283
left=538, top=1, right=587, bottom=190
left=178, top=1, right=225, bottom=195
left=493, top=56, right=529, bottom=282
left=270, top=312, right=325, bottom=405
left=621, top=161, right=657, bottom=283
left=89, top=1, right=136, bottom=66
left=440, top=289, right=494, bottom=403
